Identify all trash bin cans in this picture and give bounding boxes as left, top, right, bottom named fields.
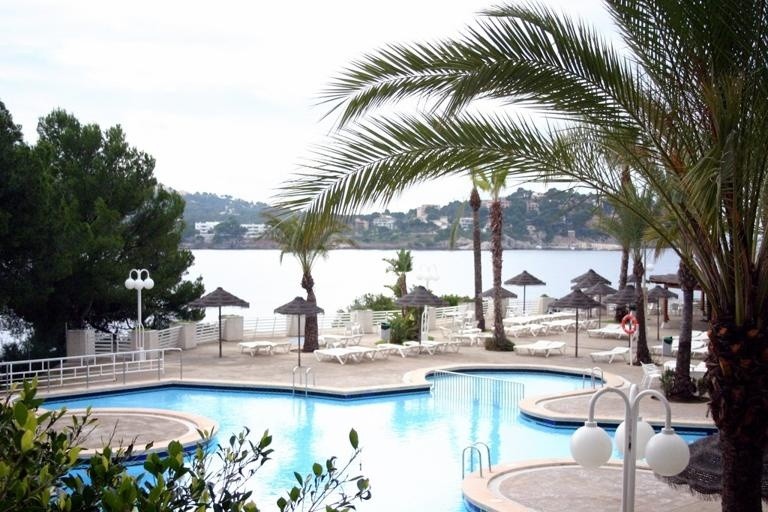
left=662, top=336, right=672, bottom=357
left=380, top=322, right=391, bottom=340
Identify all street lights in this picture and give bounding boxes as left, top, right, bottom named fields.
left=125, top=268, right=154, bottom=362
left=569, top=381, right=692, bottom=511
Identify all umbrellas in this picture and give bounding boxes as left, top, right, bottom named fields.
left=570, top=268, right=680, bottom=348
left=477, top=285, right=518, bottom=300
left=502, top=269, right=548, bottom=314
left=393, top=283, right=450, bottom=345
left=272, top=295, right=326, bottom=367
left=189, top=286, right=251, bottom=359
left=548, top=286, right=607, bottom=357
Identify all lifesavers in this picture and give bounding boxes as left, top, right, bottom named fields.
left=621, top=314, right=638, bottom=334
